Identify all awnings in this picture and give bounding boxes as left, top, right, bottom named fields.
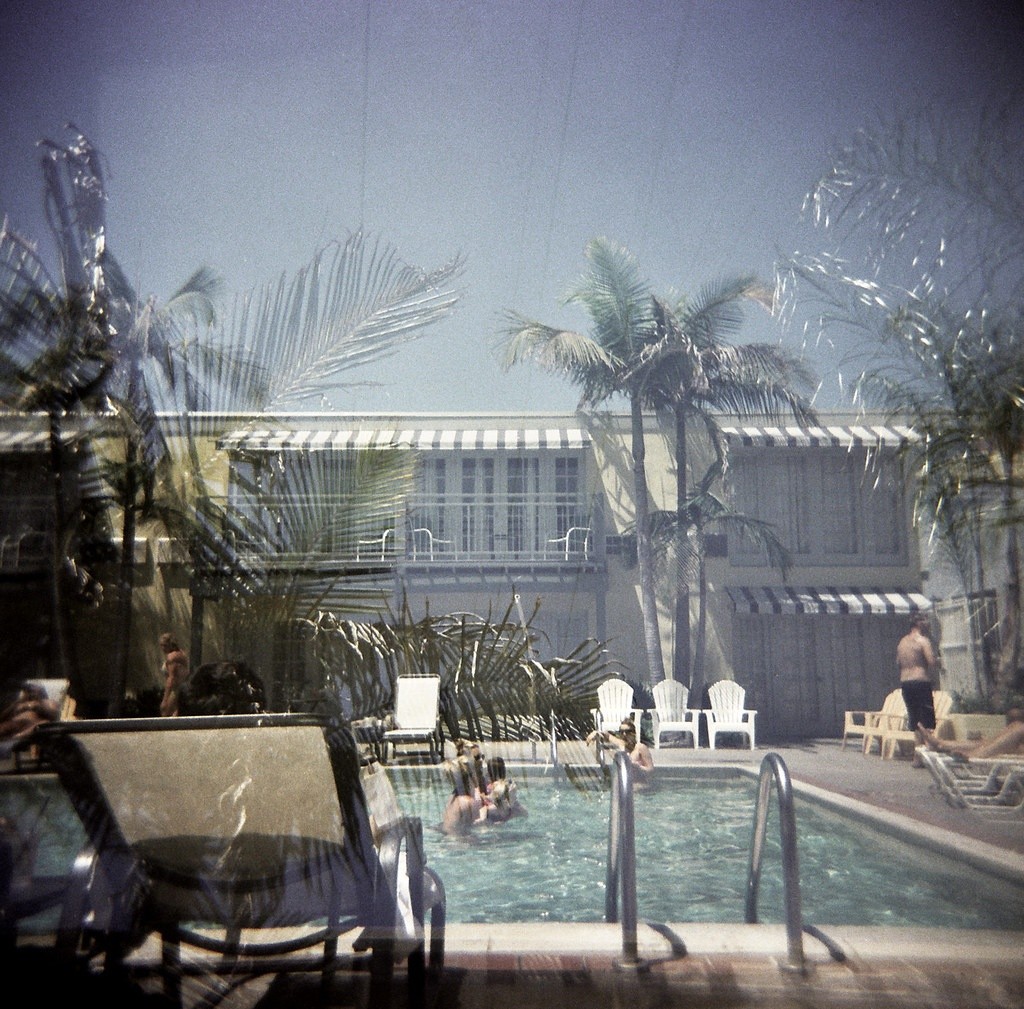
left=722, top=425, right=925, bottom=447
left=725, top=586, right=935, bottom=616
left=215, top=429, right=592, bottom=450
left=0, top=429, right=88, bottom=451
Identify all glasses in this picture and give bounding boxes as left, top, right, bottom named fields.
left=473, top=753, right=485, bottom=761
left=618, top=729, right=633, bottom=734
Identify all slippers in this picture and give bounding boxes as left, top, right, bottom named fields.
left=913, top=762, right=924, bottom=767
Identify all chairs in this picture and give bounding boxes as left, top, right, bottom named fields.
left=409, top=528, right=457, bottom=562
left=541, top=526, right=591, bottom=560
left=588, top=677, right=646, bottom=743
left=31, top=710, right=450, bottom=1009
left=647, top=676, right=701, bottom=751
left=705, top=678, right=761, bottom=750
left=354, top=527, right=395, bottom=560
left=380, top=671, right=444, bottom=765
left=839, top=684, right=1024, bottom=825
left=217, top=517, right=267, bottom=561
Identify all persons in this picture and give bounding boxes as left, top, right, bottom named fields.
left=896, top=617, right=948, bottom=767
left=159, top=633, right=189, bottom=718
left=177, top=661, right=374, bottom=951
left=917, top=668, right=1024, bottom=762
left=598, top=720, right=654, bottom=789
left=442, top=739, right=520, bottom=833
left=0, top=685, right=60, bottom=753
